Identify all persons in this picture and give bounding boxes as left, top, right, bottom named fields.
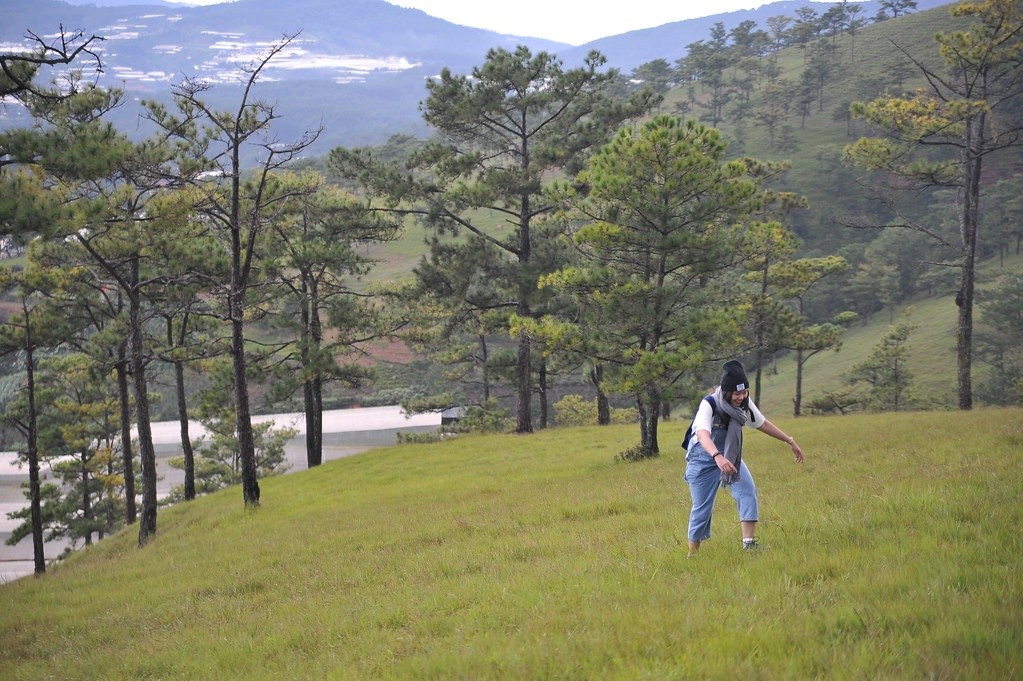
left=680, top=361, right=804, bottom=553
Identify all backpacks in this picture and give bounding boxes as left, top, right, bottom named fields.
left=682, top=395, right=717, bottom=450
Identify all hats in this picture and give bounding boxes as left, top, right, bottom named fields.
left=721, top=361, right=749, bottom=392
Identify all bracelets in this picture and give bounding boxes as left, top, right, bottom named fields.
left=788, top=436, right=793, bottom=443
left=713, top=452, right=721, bottom=459
